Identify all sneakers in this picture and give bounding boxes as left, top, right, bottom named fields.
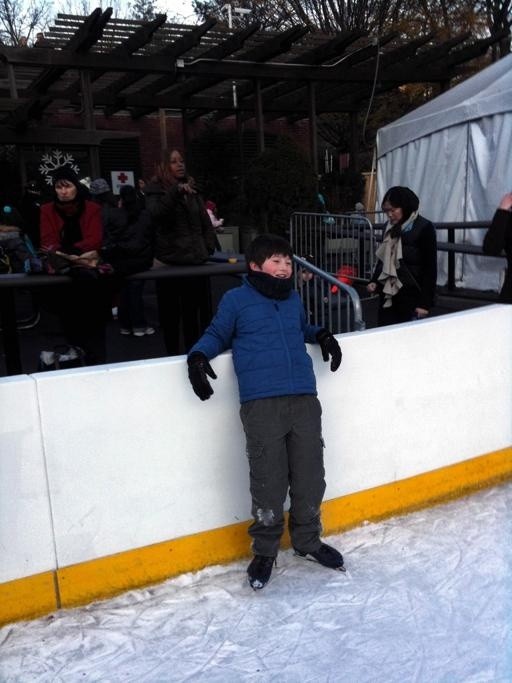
left=295, top=543, right=346, bottom=569
left=133, top=325, right=156, bottom=337
left=246, top=556, right=276, bottom=584
left=120, top=328, right=131, bottom=334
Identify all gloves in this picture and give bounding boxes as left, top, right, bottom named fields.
left=187, top=351, right=218, bottom=402
left=316, top=327, right=342, bottom=373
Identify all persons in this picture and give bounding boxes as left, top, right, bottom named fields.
left=88, top=146, right=222, bottom=359
left=482, top=189, right=511, bottom=304
left=366, top=185, right=433, bottom=327
left=39, top=166, right=101, bottom=359
left=187, top=235, right=346, bottom=593
left=3, top=180, right=45, bottom=248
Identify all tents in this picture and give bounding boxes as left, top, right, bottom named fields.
left=373, top=51, right=510, bottom=294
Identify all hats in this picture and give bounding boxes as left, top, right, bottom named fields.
left=91, top=179, right=111, bottom=195
left=52, top=165, right=80, bottom=190
